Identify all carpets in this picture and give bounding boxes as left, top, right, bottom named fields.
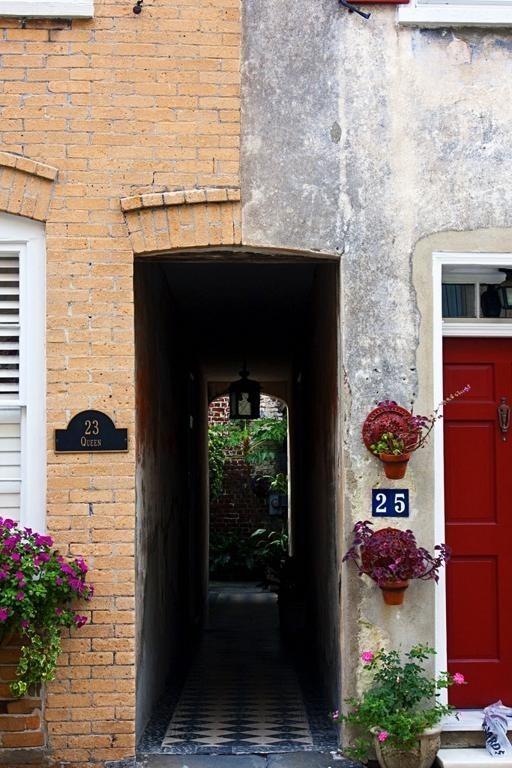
left=138, top=586, right=335, bottom=755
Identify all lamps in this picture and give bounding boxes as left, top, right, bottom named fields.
left=229, top=358, right=262, bottom=419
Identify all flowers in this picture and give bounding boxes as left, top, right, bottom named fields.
left=361, top=384, right=474, bottom=455
left=0, top=517, right=95, bottom=698
left=331, top=643, right=466, bottom=758
left=342, top=519, right=453, bottom=586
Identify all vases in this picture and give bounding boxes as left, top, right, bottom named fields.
left=379, top=452, right=411, bottom=477
left=370, top=729, right=441, bottom=768
left=379, top=580, right=408, bottom=606
